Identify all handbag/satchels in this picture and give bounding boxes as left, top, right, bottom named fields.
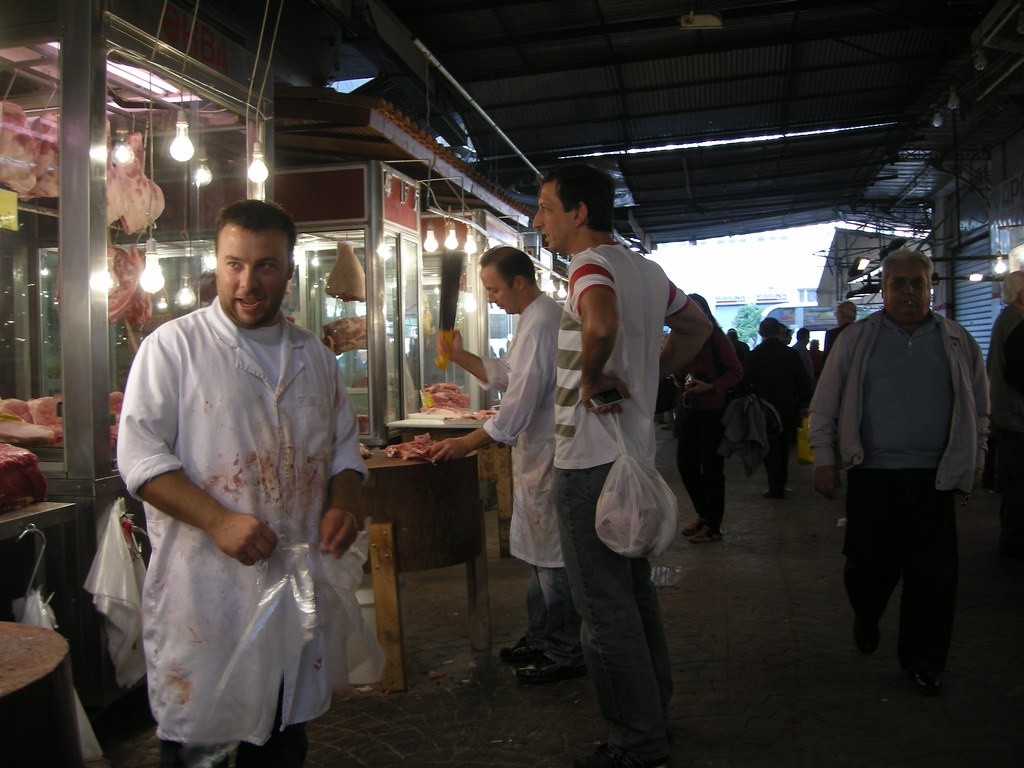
left=797, top=418, right=815, bottom=465
left=593, top=407, right=677, bottom=557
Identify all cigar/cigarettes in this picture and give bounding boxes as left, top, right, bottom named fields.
left=908, top=300, right=911, bottom=305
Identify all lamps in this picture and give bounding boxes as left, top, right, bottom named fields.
left=679, top=0, right=725, bottom=31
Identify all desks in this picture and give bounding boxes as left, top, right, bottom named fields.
left=360, top=446, right=495, bottom=699
left=385, top=408, right=515, bottom=560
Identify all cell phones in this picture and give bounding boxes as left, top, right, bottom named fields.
left=589, top=388, right=625, bottom=409
left=685, top=374, right=695, bottom=389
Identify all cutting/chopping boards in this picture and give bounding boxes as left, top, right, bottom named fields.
left=0, top=620, right=85, bottom=768
left=364, top=443, right=485, bottom=575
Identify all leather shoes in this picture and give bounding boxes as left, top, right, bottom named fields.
left=911, top=671, right=944, bottom=696
left=852, top=615, right=880, bottom=654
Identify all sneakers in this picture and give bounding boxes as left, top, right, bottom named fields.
left=514, top=654, right=588, bottom=682
left=574, top=743, right=672, bottom=768
left=499, top=635, right=544, bottom=664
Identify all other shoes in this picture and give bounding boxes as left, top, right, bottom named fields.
left=682, top=519, right=707, bottom=536
left=688, top=526, right=722, bottom=542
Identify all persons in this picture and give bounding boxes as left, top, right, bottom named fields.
left=985, top=271, right=1024, bottom=567
left=425, top=247, right=590, bottom=688
left=726, top=328, right=753, bottom=368
left=117, top=200, right=369, bottom=768
left=809, top=249, right=990, bottom=696
left=677, top=295, right=744, bottom=545
left=780, top=301, right=859, bottom=401
left=738, top=317, right=809, bottom=499
left=532, top=165, right=713, bottom=768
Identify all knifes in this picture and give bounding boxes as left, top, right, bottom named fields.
left=433, top=249, right=467, bottom=371
